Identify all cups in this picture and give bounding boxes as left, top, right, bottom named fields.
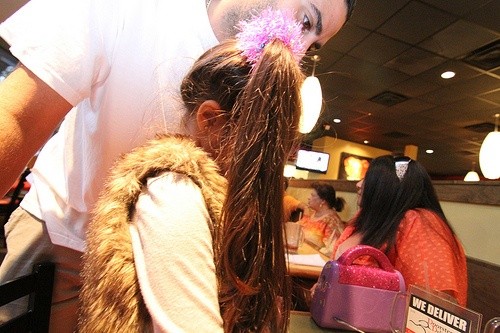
left=283, top=222, right=301, bottom=254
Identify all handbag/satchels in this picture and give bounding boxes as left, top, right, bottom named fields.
left=310, top=244, right=409, bottom=333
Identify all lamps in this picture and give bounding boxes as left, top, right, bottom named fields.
left=464, top=162, right=481, bottom=181
left=297, top=54, right=324, bottom=134
left=478, top=114, right=500, bottom=179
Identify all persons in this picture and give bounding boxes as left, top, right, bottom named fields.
left=0, top=0, right=356, bottom=333
left=312, top=154, right=469, bottom=308
left=282, top=176, right=311, bottom=221
left=78, top=11, right=305, bottom=333
left=296, top=183, right=345, bottom=259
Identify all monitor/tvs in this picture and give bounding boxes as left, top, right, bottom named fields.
left=295, top=148, right=330, bottom=174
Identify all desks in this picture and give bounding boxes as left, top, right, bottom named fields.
left=284, top=243, right=333, bottom=309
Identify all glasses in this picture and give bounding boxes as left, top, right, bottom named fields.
left=394, top=156, right=411, bottom=182
